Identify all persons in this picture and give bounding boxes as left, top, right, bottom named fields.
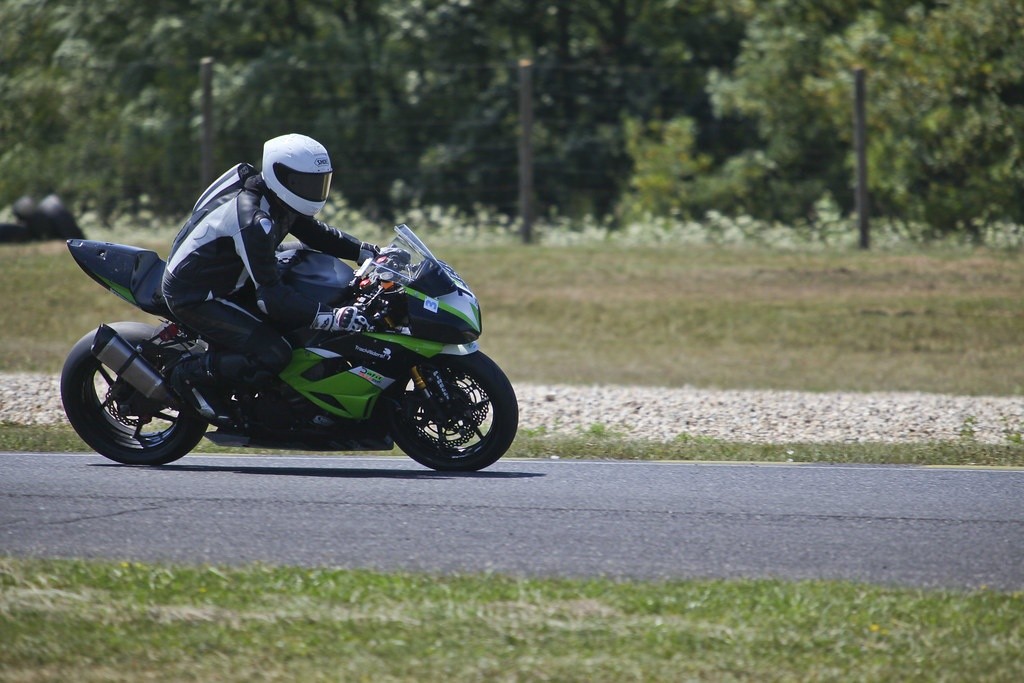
left=163, top=133, right=381, bottom=419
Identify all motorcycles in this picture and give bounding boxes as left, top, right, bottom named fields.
left=60, top=223, right=519, bottom=474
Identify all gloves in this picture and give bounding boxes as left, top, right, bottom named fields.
left=309, top=301, right=372, bottom=333
left=357, top=241, right=412, bottom=267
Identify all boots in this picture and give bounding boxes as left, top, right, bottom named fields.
left=170, top=354, right=228, bottom=422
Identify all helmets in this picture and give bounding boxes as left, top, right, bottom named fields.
left=261, top=132, right=332, bottom=215
